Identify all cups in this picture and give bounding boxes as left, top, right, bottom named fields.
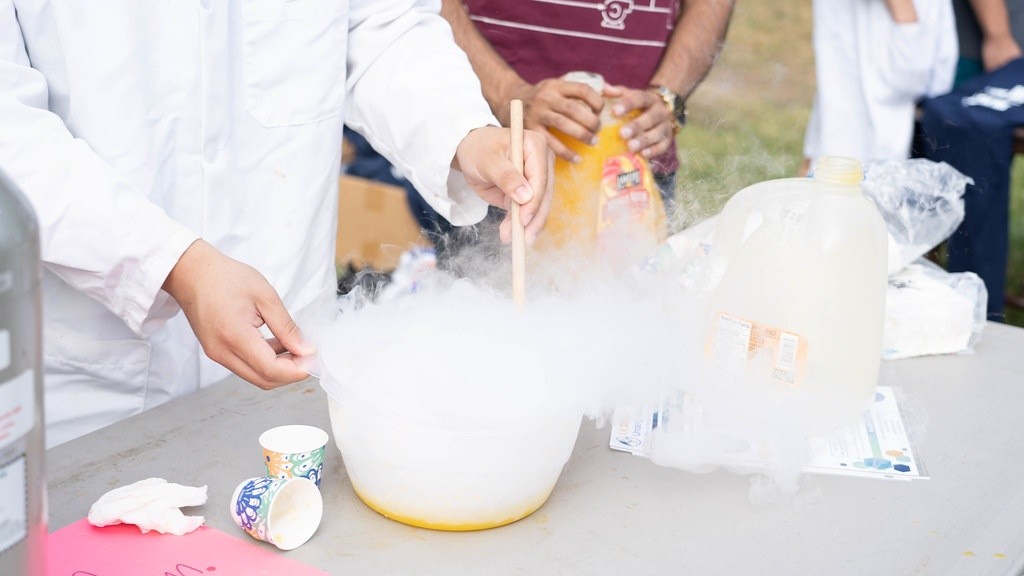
left=231, top=478, right=323, bottom=550
left=259, top=424, right=329, bottom=488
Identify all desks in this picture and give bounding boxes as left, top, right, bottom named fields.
left=44, top=321, right=1024, bottom=576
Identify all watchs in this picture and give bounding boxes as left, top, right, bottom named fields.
left=643, top=84, right=689, bottom=135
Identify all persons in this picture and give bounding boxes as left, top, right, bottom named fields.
left=0, top=0, right=557, bottom=455
left=438, top=0, right=735, bottom=235
left=798, top=0, right=1024, bottom=323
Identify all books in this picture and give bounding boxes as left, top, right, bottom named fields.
left=609, top=361, right=931, bottom=484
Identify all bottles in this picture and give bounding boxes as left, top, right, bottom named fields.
left=533, top=72, right=668, bottom=263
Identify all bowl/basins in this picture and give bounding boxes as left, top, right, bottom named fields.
left=291, top=353, right=595, bottom=532
